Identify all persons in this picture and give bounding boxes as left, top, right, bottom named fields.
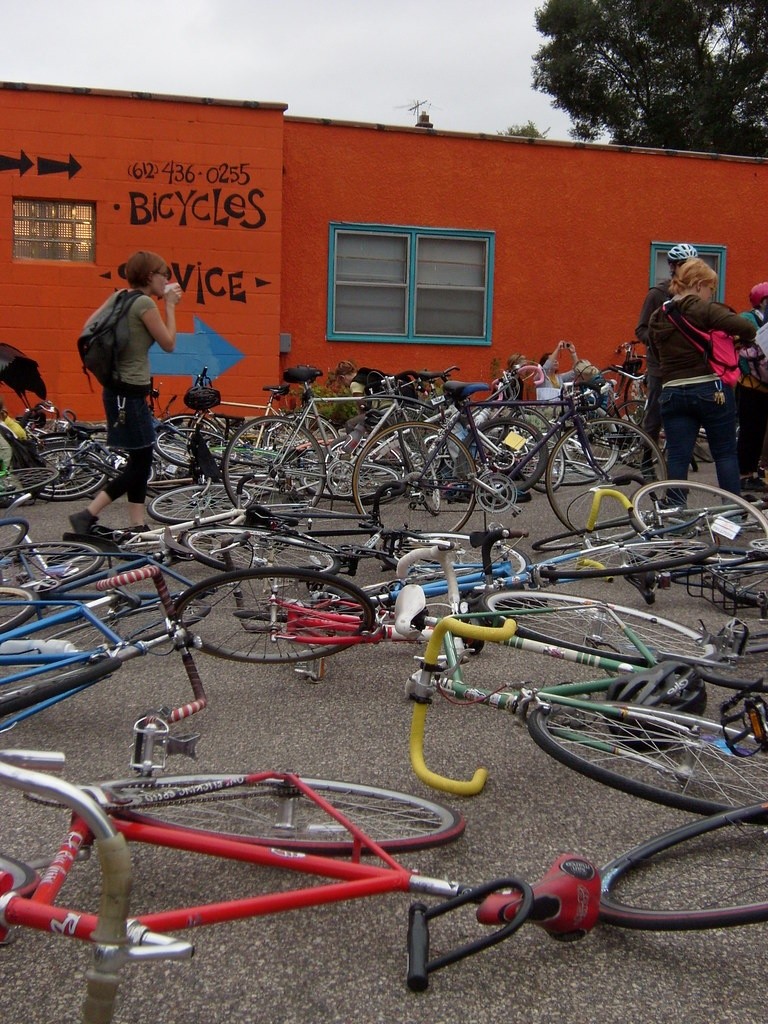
left=649, top=258, right=756, bottom=536
left=735, top=282, right=768, bottom=493
left=535, top=340, right=578, bottom=389
left=67, top=252, right=183, bottom=542
left=635, top=243, right=698, bottom=481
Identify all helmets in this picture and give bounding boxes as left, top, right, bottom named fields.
left=750, top=282, right=768, bottom=307
left=667, top=244, right=699, bottom=264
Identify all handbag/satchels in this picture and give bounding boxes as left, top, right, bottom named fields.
left=662, top=300, right=743, bottom=389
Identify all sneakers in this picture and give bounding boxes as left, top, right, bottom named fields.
left=740, top=475, right=768, bottom=494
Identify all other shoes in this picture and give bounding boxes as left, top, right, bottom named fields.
left=69, top=509, right=99, bottom=535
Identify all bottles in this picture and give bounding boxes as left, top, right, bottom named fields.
left=472, top=408, right=491, bottom=426
left=342, top=421, right=366, bottom=452
left=0, top=640, right=83, bottom=656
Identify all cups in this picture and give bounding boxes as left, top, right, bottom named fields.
left=165, top=284, right=180, bottom=304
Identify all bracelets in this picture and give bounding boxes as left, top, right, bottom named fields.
left=572, top=352, right=576, bottom=356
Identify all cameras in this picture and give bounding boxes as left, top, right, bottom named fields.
left=561, top=342, right=571, bottom=349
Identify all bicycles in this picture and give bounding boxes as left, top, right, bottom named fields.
left=0, top=340, right=768, bottom=1023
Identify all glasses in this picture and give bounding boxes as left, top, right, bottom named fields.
left=150, top=270, right=168, bottom=277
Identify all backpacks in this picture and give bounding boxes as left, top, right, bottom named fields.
left=77, top=290, right=143, bottom=374
